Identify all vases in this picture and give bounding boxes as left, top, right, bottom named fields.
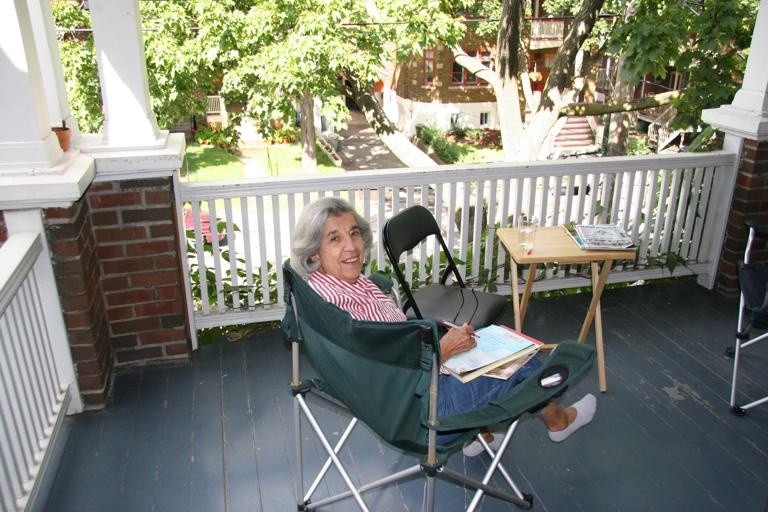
left=53, top=127, right=71, bottom=152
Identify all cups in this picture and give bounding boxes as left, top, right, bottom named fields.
left=518, top=217, right=539, bottom=249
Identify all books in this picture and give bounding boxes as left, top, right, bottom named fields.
left=439, top=322, right=544, bottom=380
left=561, top=221, right=637, bottom=251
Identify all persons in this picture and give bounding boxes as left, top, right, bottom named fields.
left=290, top=196, right=597, bottom=457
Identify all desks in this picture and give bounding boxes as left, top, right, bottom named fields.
left=497, top=227, right=635, bottom=392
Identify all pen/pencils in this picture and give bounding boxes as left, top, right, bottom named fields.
left=439, top=318, right=480, bottom=339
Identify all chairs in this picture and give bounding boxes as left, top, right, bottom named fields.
left=384, top=206, right=506, bottom=330
left=727, top=222, right=768, bottom=416
left=283, top=260, right=597, bottom=511
